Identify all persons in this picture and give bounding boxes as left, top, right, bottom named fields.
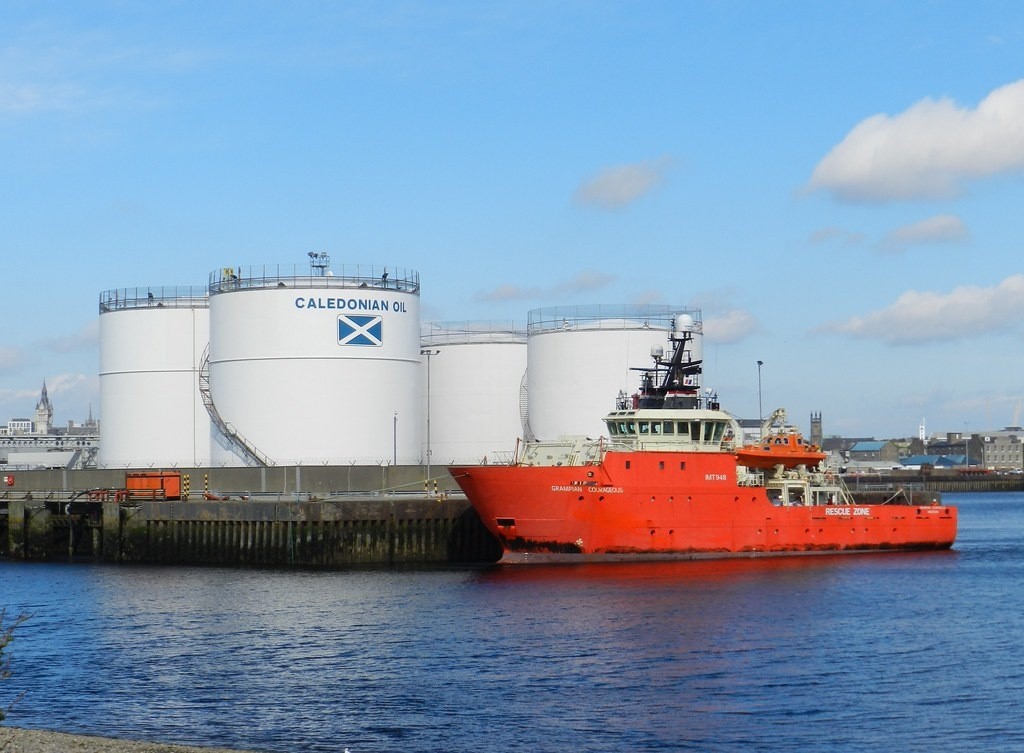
left=618, top=389, right=624, bottom=410
left=929, top=498, right=940, bottom=506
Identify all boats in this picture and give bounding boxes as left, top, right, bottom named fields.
left=448, top=313, right=958, bottom=563
